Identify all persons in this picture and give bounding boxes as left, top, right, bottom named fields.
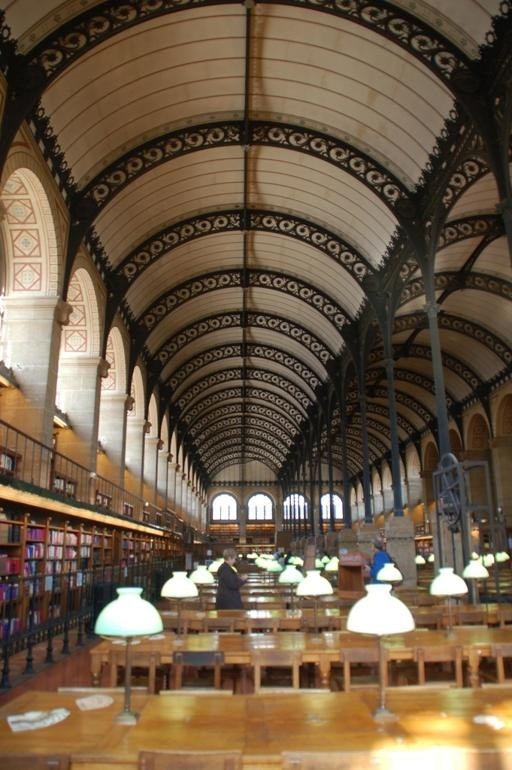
left=216, top=549, right=249, bottom=611
left=364, top=540, right=395, bottom=595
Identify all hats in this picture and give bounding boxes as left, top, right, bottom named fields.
left=370, top=538, right=382, bottom=549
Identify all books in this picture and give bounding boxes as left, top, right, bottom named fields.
left=0, top=522, right=179, bottom=658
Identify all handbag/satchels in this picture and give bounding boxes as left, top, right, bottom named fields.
left=390, top=559, right=404, bottom=586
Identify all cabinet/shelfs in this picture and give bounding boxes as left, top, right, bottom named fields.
left=0, top=503, right=188, bottom=692
